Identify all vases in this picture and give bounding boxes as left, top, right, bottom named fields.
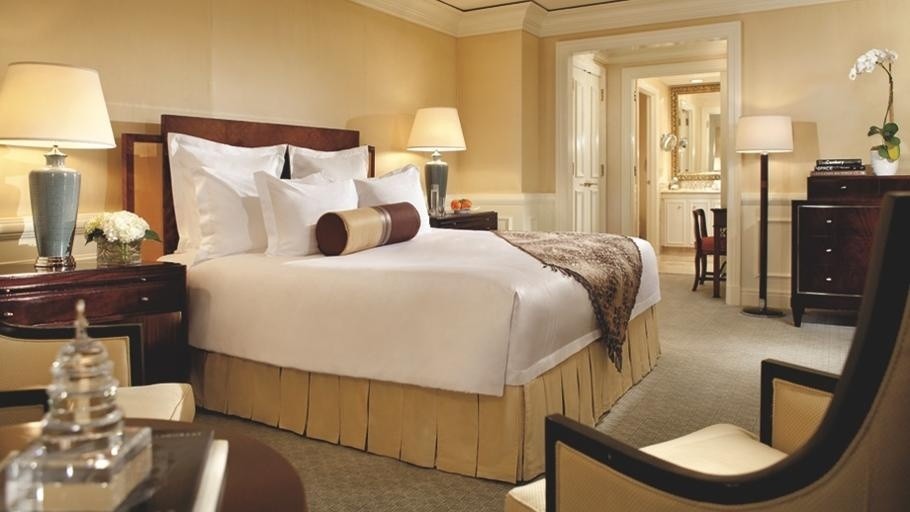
left=96, top=235, right=141, bottom=266
left=870, top=149, right=900, bottom=176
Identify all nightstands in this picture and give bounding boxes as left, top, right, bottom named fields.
left=0, top=258, right=188, bottom=421
left=428, top=209, right=498, bottom=231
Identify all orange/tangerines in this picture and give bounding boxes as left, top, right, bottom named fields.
left=450, top=200, right=461, bottom=209
left=460, top=199, right=472, bottom=208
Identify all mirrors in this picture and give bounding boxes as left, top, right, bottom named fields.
left=669, top=82, right=721, bottom=183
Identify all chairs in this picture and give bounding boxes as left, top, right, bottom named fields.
left=692, top=208, right=727, bottom=299
left=504, top=191, right=909, bottom=512
left=0, top=322, right=198, bottom=423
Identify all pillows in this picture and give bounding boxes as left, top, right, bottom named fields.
left=163, top=130, right=430, bottom=264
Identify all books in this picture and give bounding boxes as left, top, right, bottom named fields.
left=810, top=155, right=866, bottom=176
left=109, top=427, right=235, bottom=512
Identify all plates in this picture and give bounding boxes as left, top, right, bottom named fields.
left=449, top=208, right=480, bottom=214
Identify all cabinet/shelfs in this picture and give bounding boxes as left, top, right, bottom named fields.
left=790, top=175, right=910, bottom=328
left=666, top=199, right=720, bottom=247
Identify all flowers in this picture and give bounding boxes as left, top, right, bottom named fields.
left=849, top=48, right=901, bottom=161
left=82, top=209, right=162, bottom=247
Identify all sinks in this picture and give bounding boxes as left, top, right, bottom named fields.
left=712, top=180, right=721, bottom=190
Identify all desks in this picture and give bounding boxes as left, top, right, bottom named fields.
left=1, top=417, right=308, bottom=512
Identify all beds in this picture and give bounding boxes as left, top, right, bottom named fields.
left=120, top=114, right=660, bottom=481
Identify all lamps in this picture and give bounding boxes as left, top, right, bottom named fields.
left=0, top=62, right=118, bottom=268
left=406, top=107, right=467, bottom=216
left=734, top=115, right=795, bottom=318
